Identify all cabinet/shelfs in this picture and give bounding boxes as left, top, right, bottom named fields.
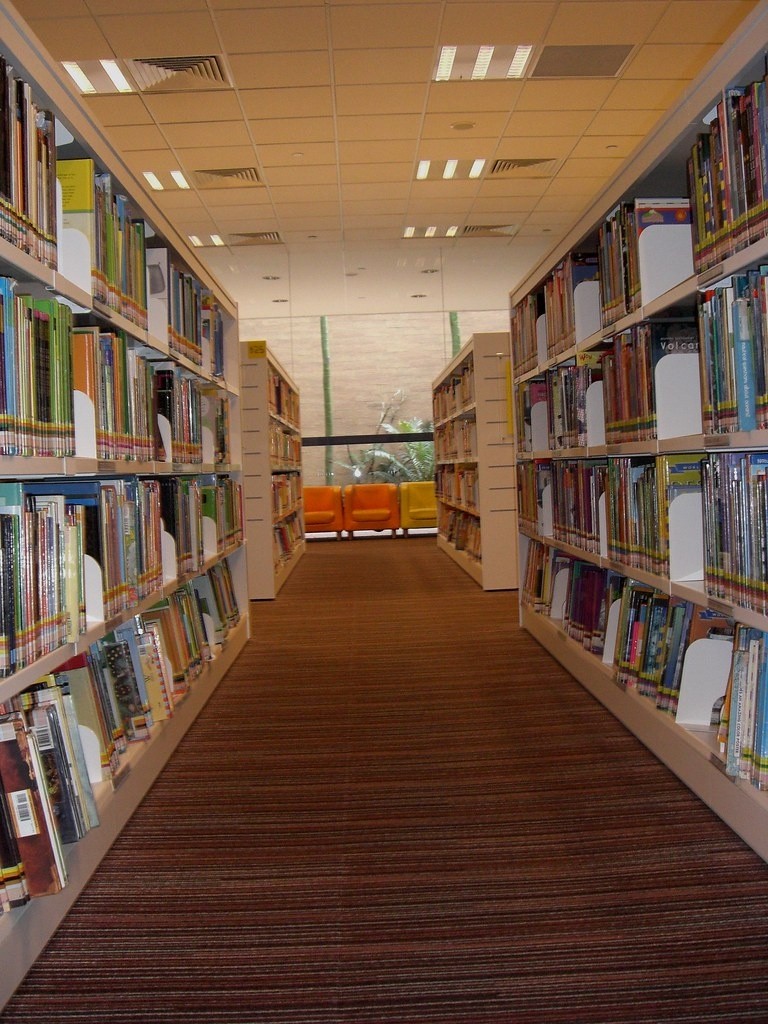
left=238, top=339, right=305, bottom=601
left=431, top=332, right=519, bottom=591
left=0, top=0, right=254, bottom=1012
left=505, top=0, right=768, bottom=869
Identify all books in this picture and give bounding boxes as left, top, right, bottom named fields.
left=434, top=364, right=475, bottom=426
left=272, top=472, right=302, bottom=514
left=273, top=511, right=304, bottom=568
left=438, top=501, right=481, bottom=562
left=519, top=539, right=768, bottom=794
left=0, top=270, right=231, bottom=466
left=434, top=464, right=479, bottom=513
left=515, top=452, right=768, bottom=618
left=0, top=557, right=240, bottom=916
left=270, top=415, right=302, bottom=468
left=509, top=48, right=768, bottom=380
left=434, top=420, right=478, bottom=462
left=267, top=359, right=300, bottom=430
left=0, top=50, right=225, bottom=382
left=513, top=262, right=768, bottom=454
left=0, top=472, right=244, bottom=679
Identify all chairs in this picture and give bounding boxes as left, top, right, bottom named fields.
left=344, top=483, right=399, bottom=541
left=303, top=486, right=344, bottom=541
left=400, top=481, right=437, bottom=539
